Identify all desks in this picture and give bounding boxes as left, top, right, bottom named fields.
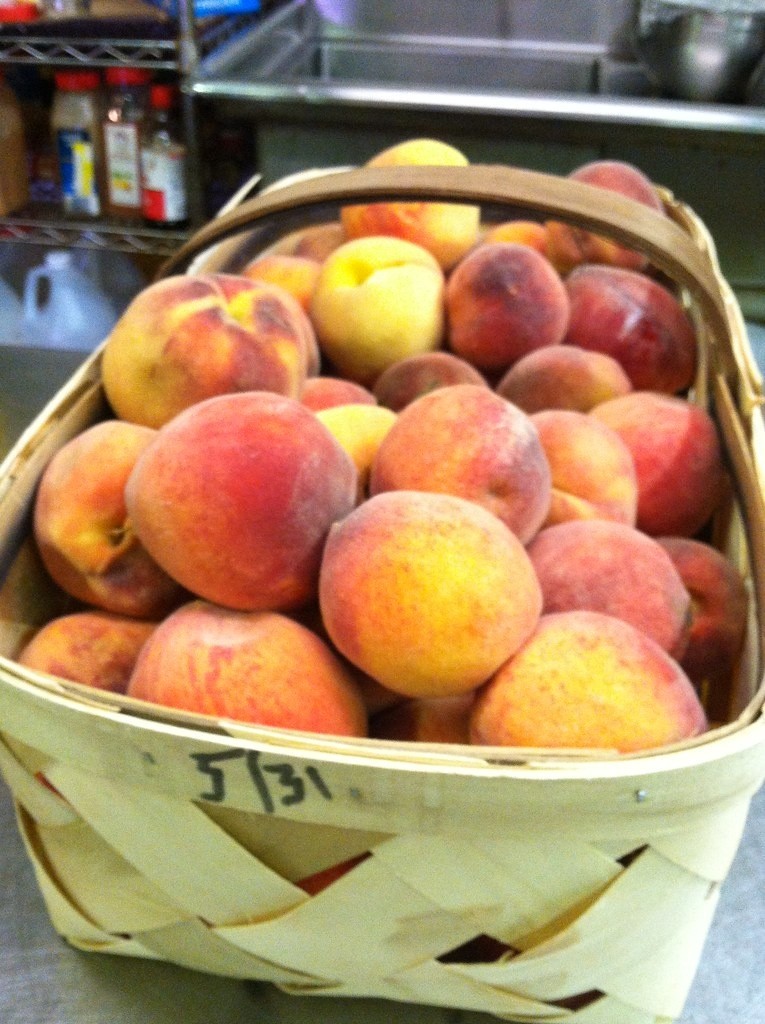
left=0, top=345, right=765, bottom=1022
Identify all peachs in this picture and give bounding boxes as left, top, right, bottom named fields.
left=14, top=139, right=749, bottom=895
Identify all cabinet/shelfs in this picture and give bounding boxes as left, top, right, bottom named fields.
left=0, top=0, right=274, bottom=255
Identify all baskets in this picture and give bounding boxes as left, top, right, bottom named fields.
left=2, top=165, right=762, bottom=1023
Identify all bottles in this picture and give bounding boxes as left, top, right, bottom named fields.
left=48, top=67, right=188, bottom=227
